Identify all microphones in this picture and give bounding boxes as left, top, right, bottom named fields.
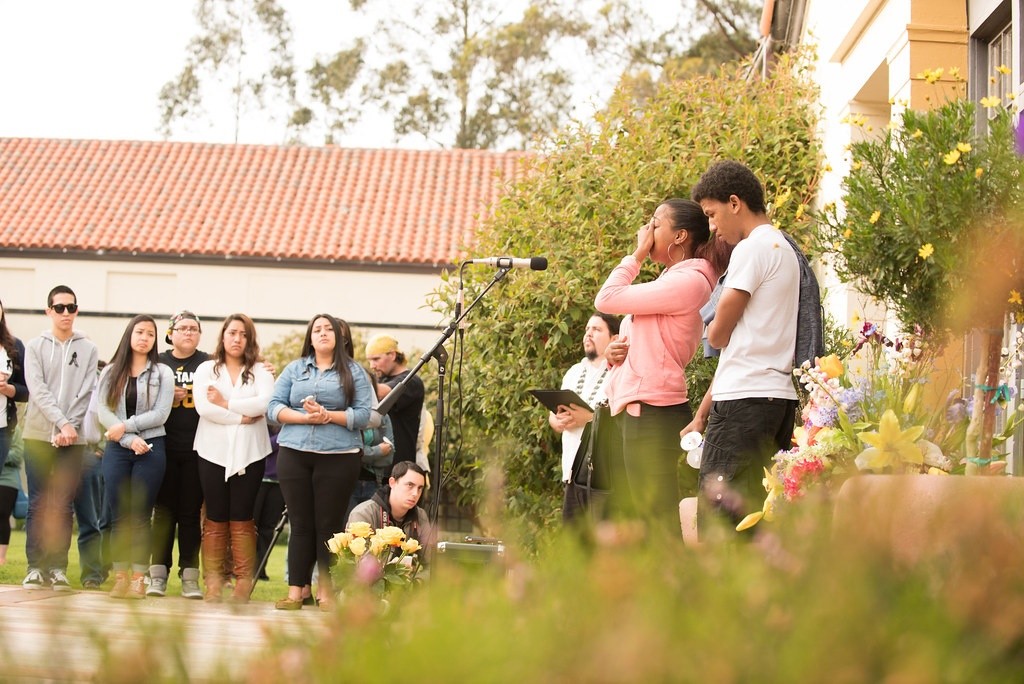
left=466, top=257, right=547, bottom=271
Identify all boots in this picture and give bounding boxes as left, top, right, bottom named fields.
left=228, top=519, right=256, bottom=605
left=201, top=518, right=230, bottom=603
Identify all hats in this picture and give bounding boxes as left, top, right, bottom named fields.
left=165, top=310, right=202, bottom=345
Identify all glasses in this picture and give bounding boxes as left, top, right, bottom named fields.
left=51, top=304, right=78, bottom=314
left=171, top=327, right=199, bottom=335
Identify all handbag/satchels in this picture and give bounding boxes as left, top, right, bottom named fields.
left=562, top=421, right=611, bottom=527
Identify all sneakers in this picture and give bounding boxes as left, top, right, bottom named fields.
left=110, top=575, right=129, bottom=597
left=143, top=564, right=170, bottom=596
left=126, top=578, right=146, bottom=599
left=180, top=567, right=203, bottom=599
left=48, top=567, right=70, bottom=592
left=22, top=569, right=44, bottom=590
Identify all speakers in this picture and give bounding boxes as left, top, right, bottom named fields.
left=437, top=542, right=507, bottom=585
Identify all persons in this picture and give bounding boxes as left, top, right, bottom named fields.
left=346, top=460, right=433, bottom=623
left=0, top=285, right=434, bottom=610
left=547, top=311, right=619, bottom=529
left=679, top=159, right=801, bottom=542
left=593, top=198, right=721, bottom=542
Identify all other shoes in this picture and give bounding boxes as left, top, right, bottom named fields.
left=275, top=596, right=300, bottom=610
left=304, top=594, right=315, bottom=605
left=319, top=597, right=334, bottom=611
left=85, top=580, right=100, bottom=591
left=101, top=563, right=113, bottom=584
